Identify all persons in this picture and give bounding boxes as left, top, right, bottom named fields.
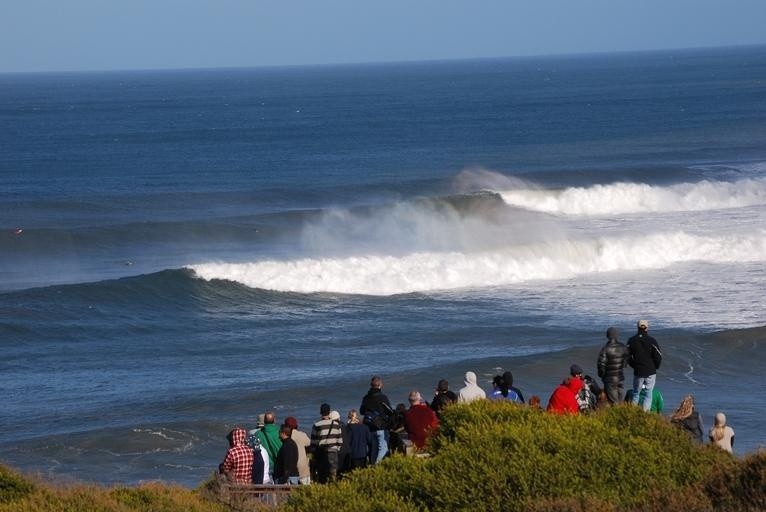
left=220, top=403, right=346, bottom=484
left=564, top=364, right=597, bottom=406
left=627, top=318, right=662, bottom=409
left=596, top=324, right=627, bottom=402
left=344, top=377, right=438, bottom=472
left=626, top=382, right=665, bottom=415
left=709, top=411, right=734, bottom=454
left=546, top=377, right=582, bottom=414
left=670, top=395, right=704, bottom=442
left=430, top=370, right=542, bottom=413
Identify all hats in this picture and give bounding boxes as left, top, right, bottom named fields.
left=715, top=413, right=726, bottom=426
left=637, top=319, right=648, bottom=329
left=285, top=416, right=298, bottom=427
left=570, top=364, right=583, bottom=377
left=257, top=414, right=264, bottom=424
left=329, top=411, right=340, bottom=421
left=606, top=327, right=618, bottom=337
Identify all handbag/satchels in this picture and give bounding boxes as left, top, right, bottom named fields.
left=311, top=446, right=329, bottom=477
